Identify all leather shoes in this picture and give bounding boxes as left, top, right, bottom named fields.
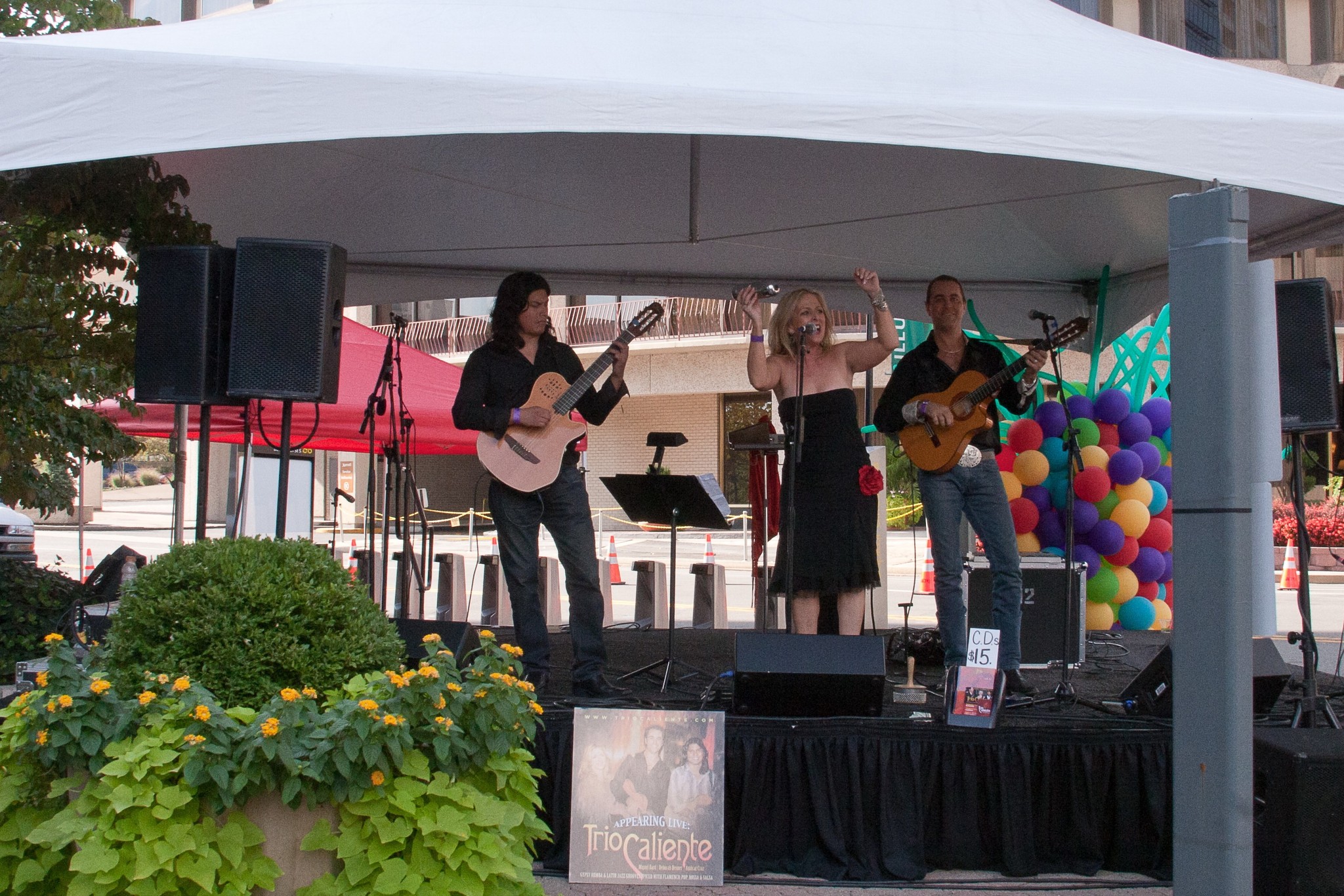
left=522, top=669, right=553, bottom=692
left=573, top=674, right=632, bottom=700
left=1003, top=669, right=1039, bottom=696
left=936, top=665, right=950, bottom=691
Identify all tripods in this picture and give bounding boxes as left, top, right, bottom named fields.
left=598, top=474, right=731, bottom=694
left=1283, top=433, right=1340, bottom=731
left=1006, top=322, right=1128, bottom=716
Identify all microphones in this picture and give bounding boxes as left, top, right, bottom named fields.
left=390, top=311, right=409, bottom=328
left=334, top=487, right=355, bottom=503
left=799, top=322, right=816, bottom=333
left=1028, top=309, right=1055, bottom=322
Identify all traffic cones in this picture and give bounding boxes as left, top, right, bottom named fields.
left=608, top=535, right=625, bottom=584
left=704, top=535, right=717, bottom=565
left=84, top=549, right=97, bottom=586
left=491, top=537, right=499, bottom=557
left=1277, top=539, right=1299, bottom=590
left=913, top=539, right=936, bottom=594
left=346, top=539, right=360, bottom=588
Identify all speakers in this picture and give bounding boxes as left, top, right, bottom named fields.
left=1116, top=636, right=1292, bottom=724
left=386, top=616, right=485, bottom=684
left=734, top=630, right=887, bottom=716
left=84, top=545, right=147, bottom=603
left=227, top=235, right=349, bottom=403
left=133, top=245, right=246, bottom=407
left=1276, top=276, right=1342, bottom=436
left=1247, top=726, right=1343, bottom=895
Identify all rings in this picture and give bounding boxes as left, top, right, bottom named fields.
left=1033, top=359, right=1038, bottom=363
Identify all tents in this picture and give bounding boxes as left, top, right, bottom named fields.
left=78, top=313, right=589, bottom=583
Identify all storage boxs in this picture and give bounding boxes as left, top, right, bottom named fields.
left=961, top=550, right=1088, bottom=668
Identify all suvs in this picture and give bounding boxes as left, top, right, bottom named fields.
left=0, top=501, right=37, bottom=569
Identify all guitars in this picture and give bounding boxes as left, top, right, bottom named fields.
left=898, top=310, right=1096, bottom=475
left=475, top=301, right=666, bottom=493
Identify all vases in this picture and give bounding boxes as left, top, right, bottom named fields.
left=66, top=765, right=342, bottom=896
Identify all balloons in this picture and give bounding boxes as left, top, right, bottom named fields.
left=960, top=294, right=1173, bottom=633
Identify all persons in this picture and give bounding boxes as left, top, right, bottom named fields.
left=577, top=743, right=633, bottom=823
left=871, top=276, right=1047, bottom=698
left=663, top=737, right=720, bottom=827
left=735, top=266, right=901, bottom=636
left=450, top=272, right=632, bottom=699
left=609, top=723, right=670, bottom=821
left=964, top=686, right=993, bottom=705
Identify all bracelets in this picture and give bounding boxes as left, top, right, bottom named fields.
left=871, top=288, right=888, bottom=311
left=512, top=407, right=521, bottom=425
left=750, top=333, right=764, bottom=342
left=1021, top=376, right=1037, bottom=389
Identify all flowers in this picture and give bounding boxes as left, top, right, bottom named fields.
left=0, top=627, right=554, bottom=896
left=859, top=465, right=884, bottom=496
